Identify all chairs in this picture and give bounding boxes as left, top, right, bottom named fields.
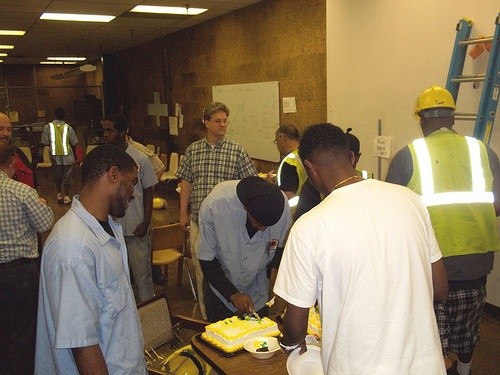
left=86, top=144, right=98, bottom=157
left=147, top=144, right=156, bottom=152
left=18, top=147, right=32, bottom=163
left=136, top=296, right=212, bottom=375
left=149, top=220, right=188, bottom=289
left=37, top=146, right=52, bottom=180
left=157, top=151, right=184, bottom=199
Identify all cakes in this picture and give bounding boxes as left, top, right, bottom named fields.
left=201, top=315, right=281, bottom=354
left=306, top=304, right=322, bottom=339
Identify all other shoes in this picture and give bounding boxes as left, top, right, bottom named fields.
left=56, top=192, right=62, bottom=200
left=63, top=195, right=72, bottom=205
left=447, top=360, right=471, bottom=375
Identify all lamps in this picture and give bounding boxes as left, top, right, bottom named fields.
left=50, top=61, right=97, bottom=80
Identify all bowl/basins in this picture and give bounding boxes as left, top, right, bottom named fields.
left=244, top=336, right=280, bottom=359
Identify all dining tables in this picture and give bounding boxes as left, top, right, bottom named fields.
left=189, top=312, right=292, bottom=375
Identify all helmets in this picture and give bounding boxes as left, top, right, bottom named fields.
left=414, top=84, right=457, bottom=114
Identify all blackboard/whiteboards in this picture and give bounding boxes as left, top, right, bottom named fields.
left=212, top=82, right=282, bottom=162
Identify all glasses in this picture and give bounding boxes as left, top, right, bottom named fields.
left=215, top=118, right=227, bottom=125
left=272, top=136, right=283, bottom=145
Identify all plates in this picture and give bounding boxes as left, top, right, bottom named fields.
left=287, top=344, right=325, bottom=375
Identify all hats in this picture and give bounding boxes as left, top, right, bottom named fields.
left=236, top=175, right=285, bottom=227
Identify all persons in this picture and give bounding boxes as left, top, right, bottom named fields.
left=0, top=103, right=361, bottom=375
left=386, top=86, right=500, bottom=375
left=272, top=122, right=449, bottom=375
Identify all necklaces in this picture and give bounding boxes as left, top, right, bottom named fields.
left=331, top=175, right=361, bottom=191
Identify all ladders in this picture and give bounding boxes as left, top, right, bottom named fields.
left=445, top=12, right=500, bottom=148
